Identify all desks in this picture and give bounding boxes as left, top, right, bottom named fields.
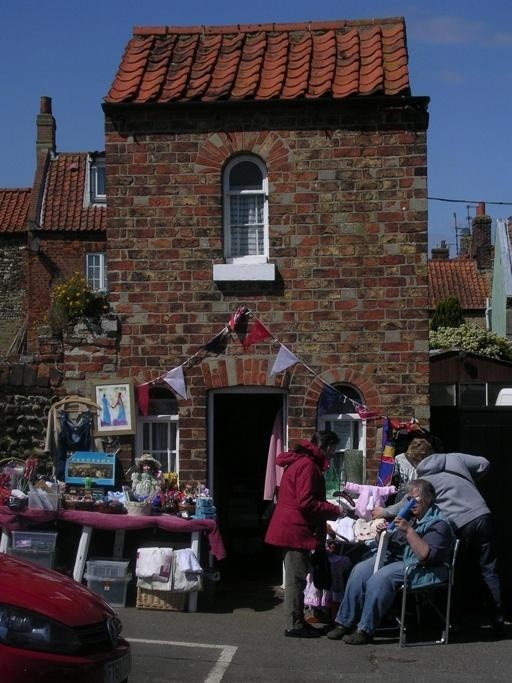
left=0, top=503, right=216, bottom=612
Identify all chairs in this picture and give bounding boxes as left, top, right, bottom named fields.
left=390, top=537, right=461, bottom=648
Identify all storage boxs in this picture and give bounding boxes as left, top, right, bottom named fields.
left=6, top=526, right=58, bottom=573
left=84, top=555, right=133, bottom=609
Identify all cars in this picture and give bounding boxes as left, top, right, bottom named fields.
left=0, top=554, right=132, bottom=682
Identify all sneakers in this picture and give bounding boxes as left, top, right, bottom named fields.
left=343, top=630, right=376, bottom=645
left=327, top=625, right=350, bottom=640
left=284, top=623, right=327, bottom=637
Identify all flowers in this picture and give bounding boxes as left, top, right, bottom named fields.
left=45, top=269, right=109, bottom=332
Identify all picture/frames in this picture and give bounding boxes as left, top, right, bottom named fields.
left=90, top=379, right=137, bottom=436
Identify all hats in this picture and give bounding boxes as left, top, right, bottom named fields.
left=133, top=452, right=162, bottom=470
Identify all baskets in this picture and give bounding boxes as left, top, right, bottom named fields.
left=135, top=583, right=186, bottom=612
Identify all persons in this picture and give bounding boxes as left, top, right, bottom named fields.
left=371, top=437, right=512, bottom=628
left=325, top=478, right=454, bottom=645
left=262, top=429, right=349, bottom=638
left=100, top=393, right=112, bottom=426
left=129, top=452, right=161, bottom=502
left=113, top=391, right=126, bottom=422
